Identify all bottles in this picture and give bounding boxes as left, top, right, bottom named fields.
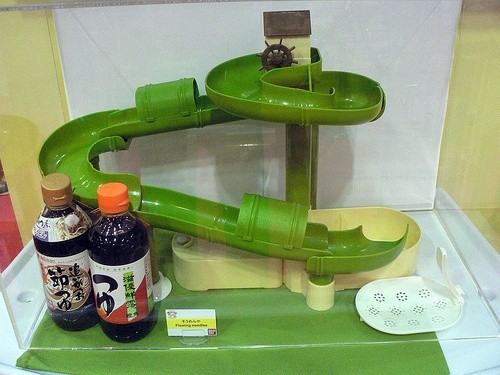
left=85, top=181, right=159, bottom=344
left=31, top=173, right=100, bottom=332
